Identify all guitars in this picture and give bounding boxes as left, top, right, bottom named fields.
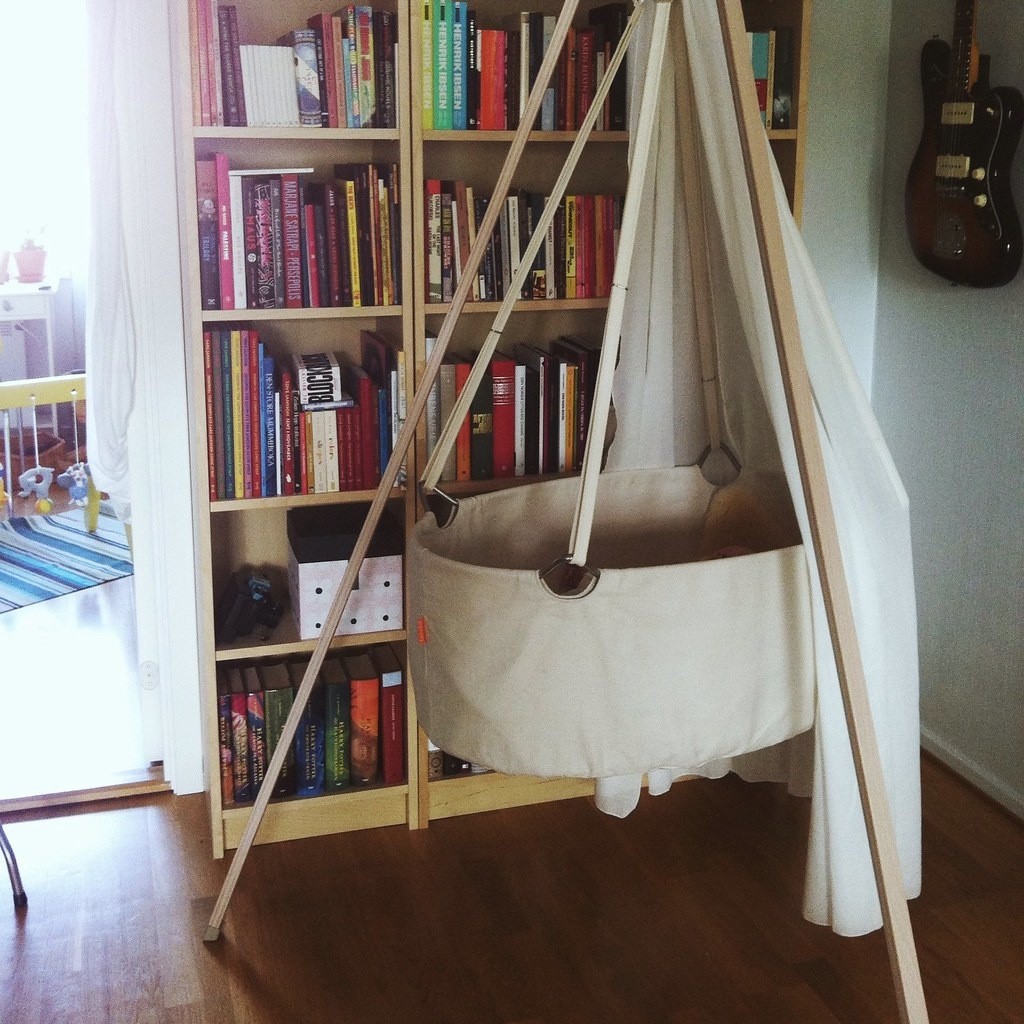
left=903, top=1, right=1024, bottom=290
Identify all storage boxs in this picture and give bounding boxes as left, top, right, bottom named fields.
left=286, top=509, right=403, bottom=641
left=0, top=432, right=65, bottom=490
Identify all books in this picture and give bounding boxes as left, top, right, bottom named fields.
left=186, top=0, right=776, bottom=806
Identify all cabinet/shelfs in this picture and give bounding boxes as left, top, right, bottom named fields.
left=0, top=282, right=59, bottom=436
left=168, top=1, right=811, bottom=862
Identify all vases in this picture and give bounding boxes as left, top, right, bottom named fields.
left=13, top=249, right=47, bottom=283
